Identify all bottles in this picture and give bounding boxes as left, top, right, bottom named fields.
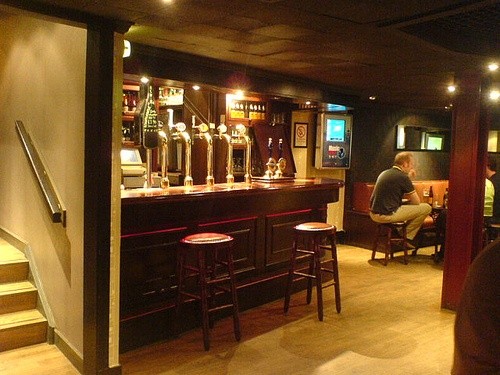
left=428, top=186, right=433, bottom=207
left=122, top=91, right=136, bottom=141
left=226, top=127, right=246, bottom=172
left=231, top=101, right=266, bottom=119
left=143, top=84, right=159, bottom=149
left=443, top=188, right=448, bottom=208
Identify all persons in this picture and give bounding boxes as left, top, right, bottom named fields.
left=432, top=153, right=500, bottom=262
left=369, top=151, right=431, bottom=250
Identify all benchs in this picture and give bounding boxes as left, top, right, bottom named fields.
left=352, top=181, right=450, bottom=254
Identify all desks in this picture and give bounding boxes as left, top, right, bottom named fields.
left=430, top=206, right=447, bottom=264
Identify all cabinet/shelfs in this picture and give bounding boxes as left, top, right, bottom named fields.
left=121, top=84, right=157, bottom=190
left=182, top=86, right=270, bottom=185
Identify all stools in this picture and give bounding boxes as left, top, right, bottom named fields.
left=284, top=222, right=340, bottom=321
left=485, top=224, right=500, bottom=244
left=371, top=222, right=408, bottom=266
left=174, top=232, right=241, bottom=353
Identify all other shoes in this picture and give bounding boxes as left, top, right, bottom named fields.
left=425, top=253, right=444, bottom=265
left=391, top=237, right=416, bottom=251
